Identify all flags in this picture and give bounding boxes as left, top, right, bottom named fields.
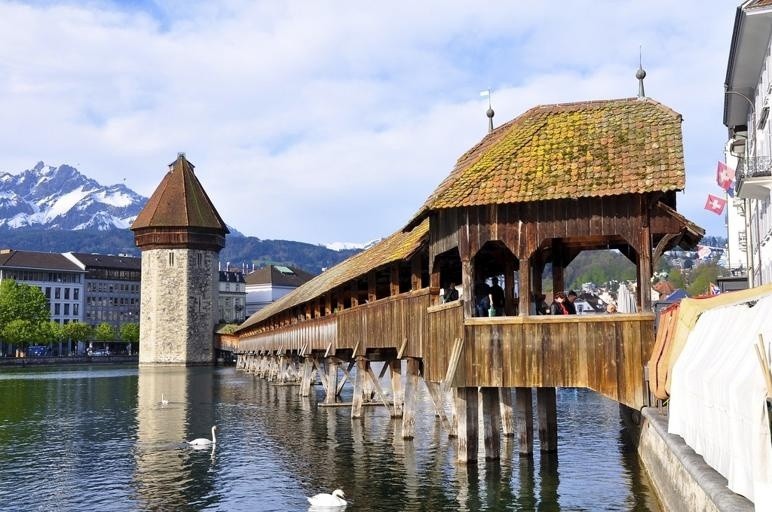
left=716, top=163, right=736, bottom=193
left=705, top=194, right=725, bottom=215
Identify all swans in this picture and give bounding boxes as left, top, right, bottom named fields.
left=186, top=424, right=220, bottom=445
left=304, top=489, right=349, bottom=508
left=161, top=393, right=168, bottom=404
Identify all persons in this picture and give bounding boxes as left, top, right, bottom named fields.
left=435, top=273, right=580, bottom=314
left=602, top=305, right=617, bottom=314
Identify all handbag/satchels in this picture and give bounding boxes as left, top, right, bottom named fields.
left=487, top=306, right=496, bottom=317
